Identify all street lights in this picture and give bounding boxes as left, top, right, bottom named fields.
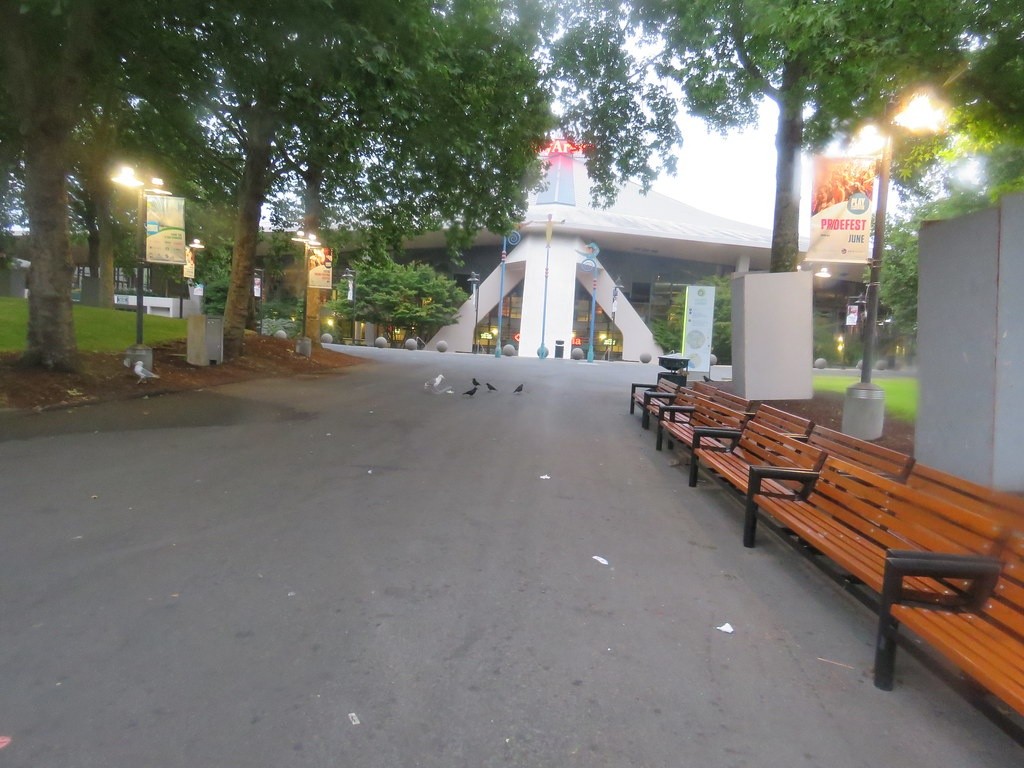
left=179, top=237, right=204, bottom=319
left=466, top=271, right=480, bottom=354
left=110, top=165, right=173, bottom=373
left=839, top=89, right=949, bottom=441
left=291, top=231, right=322, bottom=357
left=341, top=268, right=357, bottom=346
left=610, top=284, right=625, bottom=360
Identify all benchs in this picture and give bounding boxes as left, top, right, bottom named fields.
left=630, top=378, right=1024, bottom=768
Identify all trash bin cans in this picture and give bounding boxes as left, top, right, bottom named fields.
left=656, top=354, right=691, bottom=387
left=554, top=340, right=565, bottom=358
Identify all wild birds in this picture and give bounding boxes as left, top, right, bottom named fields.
left=424, top=374, right=524, bottom=396
left=134, top=361, right=160, bottom=384
